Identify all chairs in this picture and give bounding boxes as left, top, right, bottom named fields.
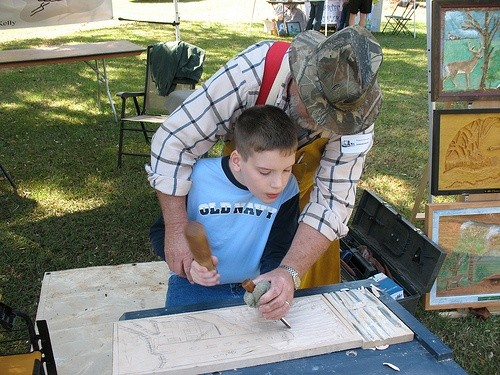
left=0, top=309, right=57, bottom=375
left=382, top=1, right=410, bottom=35
left=117, top=40, right=206, bottom=166
left=392, top=3, right=419, bottom=37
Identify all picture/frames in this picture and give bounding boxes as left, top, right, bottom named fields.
left=431, top=107, right=500, bottom=196
left=431, top=0, right=500, bottom=101
left=276, top=21, right=286, bottom=37
left=423, top=201, right=500, bottom=311
left=284, top=21, right=301, bottom=37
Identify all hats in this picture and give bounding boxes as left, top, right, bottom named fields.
left=288, top=25, right=383, bottom=136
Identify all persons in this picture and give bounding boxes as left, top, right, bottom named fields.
left=148, top=104, right=301, bottom=313
left=148, top=25, right=384, bottom=321
left=336, top=0, right=372, bottom=31
left=306, top=0, right=326, bottom=32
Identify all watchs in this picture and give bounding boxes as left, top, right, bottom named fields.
left=277, top=264, right=302, bottom=292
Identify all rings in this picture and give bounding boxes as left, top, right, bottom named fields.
left=284, top=301, right=290, bottom=307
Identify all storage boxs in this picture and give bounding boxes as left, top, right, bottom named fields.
left=342, top=189, right=447, bottom=315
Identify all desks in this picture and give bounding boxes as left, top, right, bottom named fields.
left=267, top=1, right=305, bottom=20
left=118, top=281, right=468, bottom=375
left=0, top=40, right=147, bottom=122
